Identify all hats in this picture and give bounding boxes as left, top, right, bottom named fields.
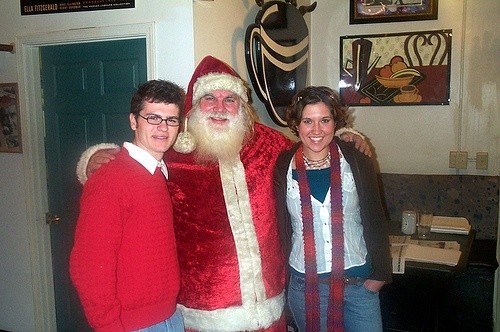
left=172, top=55, right=249, bottom=154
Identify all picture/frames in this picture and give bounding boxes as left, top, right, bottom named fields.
left=349, top=0, right=438, bottom=24
left=339, top=29, right=450, bottom=106
left=0, top=82, right=23, bottom=154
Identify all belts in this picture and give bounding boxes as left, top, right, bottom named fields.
left=318, top=276, right=357, bottom=285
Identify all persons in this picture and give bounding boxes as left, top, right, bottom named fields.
left=273, top=86, right=390, bottom=332
left=69, top=79, right=186, bottom=332
left=76, top=55, right=372, bottom=332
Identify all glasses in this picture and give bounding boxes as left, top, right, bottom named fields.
left=137, top=113, right=180, bottom=127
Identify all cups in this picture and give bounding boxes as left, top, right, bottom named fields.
left=418, top=211, right=433, bottom=240
left=401, top=210, right=416, bottom=234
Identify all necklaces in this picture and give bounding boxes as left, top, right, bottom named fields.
left=303, top=152, right=332, bottom=168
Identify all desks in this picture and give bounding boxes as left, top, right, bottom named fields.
left=390, top=221, right=477, bottom=276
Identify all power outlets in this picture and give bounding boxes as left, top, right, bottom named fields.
left=448, top=151, right=468, bottom=169
